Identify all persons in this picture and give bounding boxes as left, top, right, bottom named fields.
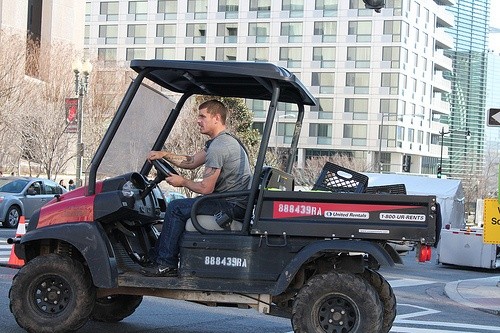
left=59, top=179, right=76, bottom=192
left=141, top=99, right=254, bottom=276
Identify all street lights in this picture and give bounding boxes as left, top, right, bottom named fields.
left=72, top=69, right=89, bottom=190
left=437, top=126, right=471, bottom=179
left=377, top=111, right=426, bottom=173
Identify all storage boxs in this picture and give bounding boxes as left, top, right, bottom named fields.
left=312, top=162, right=369, bottom=193
left=366, top=183, right=407, bottom=194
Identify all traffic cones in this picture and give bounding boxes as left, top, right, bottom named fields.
left=0, top=216, right=25, bottom=268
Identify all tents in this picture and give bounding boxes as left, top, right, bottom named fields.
left=327, top=173, right=467, bottom=239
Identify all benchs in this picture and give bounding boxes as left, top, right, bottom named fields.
left=183, top=164, right=293, bottom=234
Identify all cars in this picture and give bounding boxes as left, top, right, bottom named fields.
left=8, top=59, right=443, bottom=333
left=0, top=177, right=70, bottom=228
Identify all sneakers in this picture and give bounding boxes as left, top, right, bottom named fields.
left=136, top=254, right=178, bottom=277
